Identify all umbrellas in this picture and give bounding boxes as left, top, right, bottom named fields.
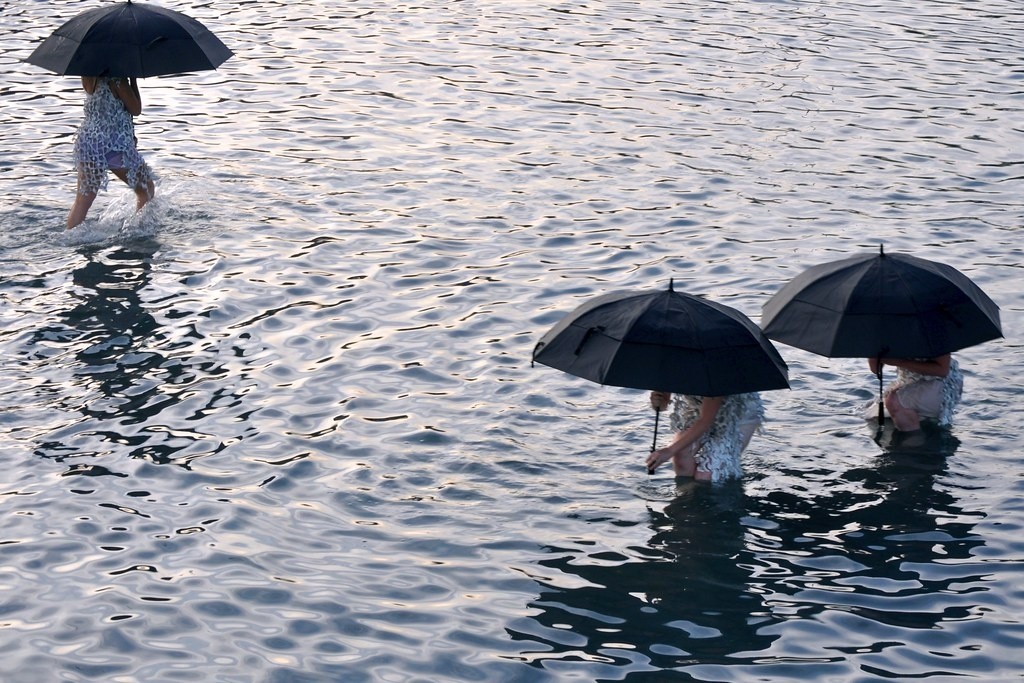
left=531, top=278, right=792, bottom=475
left=24, top=0, right=235, bottom=147
left=760, top=243, right=1004, bottom=425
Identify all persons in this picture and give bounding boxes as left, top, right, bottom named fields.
left=869, top=353, right=951, bottom=432
left=67, top=75, right=155, bottom=230
left=646, top=391, right=768, bottom=482
